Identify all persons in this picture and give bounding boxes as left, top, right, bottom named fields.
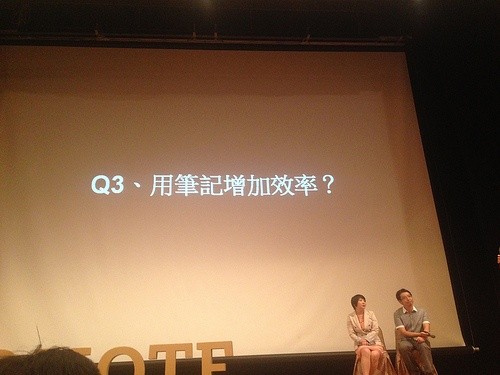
left=393, top=288, right=438, bottom=375
left=347, top=293, right=385, bottom=375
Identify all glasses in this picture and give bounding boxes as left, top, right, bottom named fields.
left=400, top=294, right=412, bottom=301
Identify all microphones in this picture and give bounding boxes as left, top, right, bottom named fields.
left=421, top=330, right=435, bottom=338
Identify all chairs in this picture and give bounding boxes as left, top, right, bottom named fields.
left=394, top=325, right=438, bottom=375
left=354, top=326, right=397, bottom=375
